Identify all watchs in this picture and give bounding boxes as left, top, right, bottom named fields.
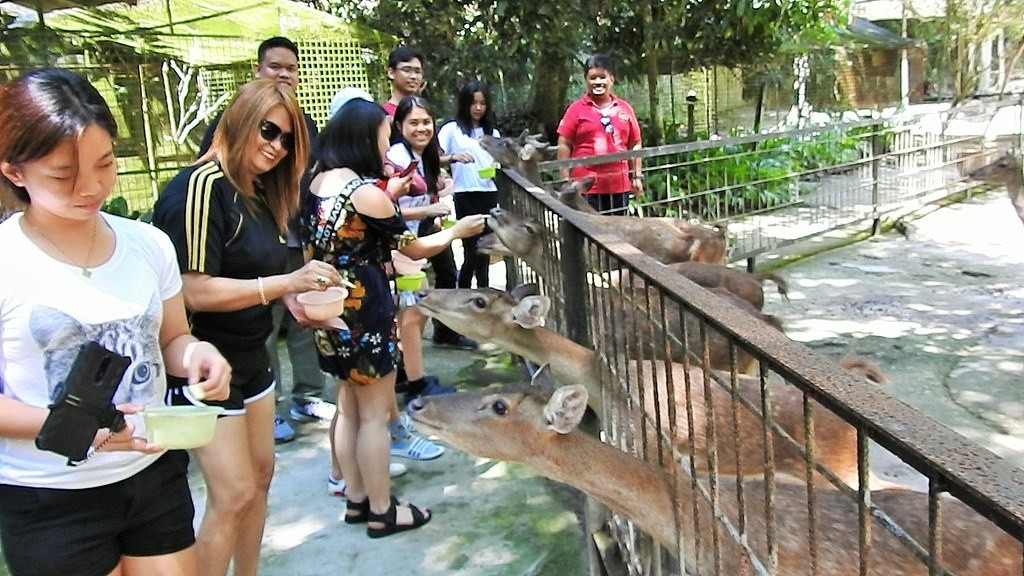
left=632, top=174, right=645, bottom=181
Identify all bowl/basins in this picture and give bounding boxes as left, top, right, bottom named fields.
left=396, top=270, right=426, bottom=291
left=136, top=405, right=225, bottom=451
left=296, top=287, right=349, bottom=321
left=478, top=167, right=496, bottom=178
left=443, top=219, right=458, bottom=229
left=390, top=249, right=428, bottom=277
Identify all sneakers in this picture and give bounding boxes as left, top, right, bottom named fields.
left=290, top=397, right=336, bottom=423
left=273, top=414, right=295, bottom=443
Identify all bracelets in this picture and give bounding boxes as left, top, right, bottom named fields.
left=257, top=277, right=269, bottom=306
left=183, top=341, right=205, bottom=368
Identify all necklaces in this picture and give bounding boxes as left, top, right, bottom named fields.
left=23, top=207, right=98, bottom=280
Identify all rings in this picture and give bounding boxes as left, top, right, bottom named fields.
left=316, top=276, right=325, bottom=284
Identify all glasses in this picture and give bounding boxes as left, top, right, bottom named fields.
left=398, top=67, right=423, bottom=74
left=260, top=118, right=295, bottom=152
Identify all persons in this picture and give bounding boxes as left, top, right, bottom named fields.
left=0, top=65, right=233, bottom=576
left=153, top=78, right=345, bottom=576
left=197, top=36, right=338, bottom=444
left=556, top=56, right=645, bottom=215
left=302, top=48, right=502, bottom=540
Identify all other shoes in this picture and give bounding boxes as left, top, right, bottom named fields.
left=326, top=475, right=349, bottom=497
left=394, top=375, right=457, bottom=406
left=389, top=462, right=408, bottom=477
left=431, top=333, right=479, bottom=351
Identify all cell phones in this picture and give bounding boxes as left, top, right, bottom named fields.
left=34, top=340, right=133, bottom=461
left=399, top=159, right=418, bottom=178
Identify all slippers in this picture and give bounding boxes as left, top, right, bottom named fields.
left=388, top=416, right=445, bottom=458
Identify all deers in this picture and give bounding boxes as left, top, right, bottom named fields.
left=969, top=148, right=1024, bottom=223
left=403, top=206, right=1024, bottom=576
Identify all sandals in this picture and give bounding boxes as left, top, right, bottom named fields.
left=344, top=496, right=432, bottom=538
